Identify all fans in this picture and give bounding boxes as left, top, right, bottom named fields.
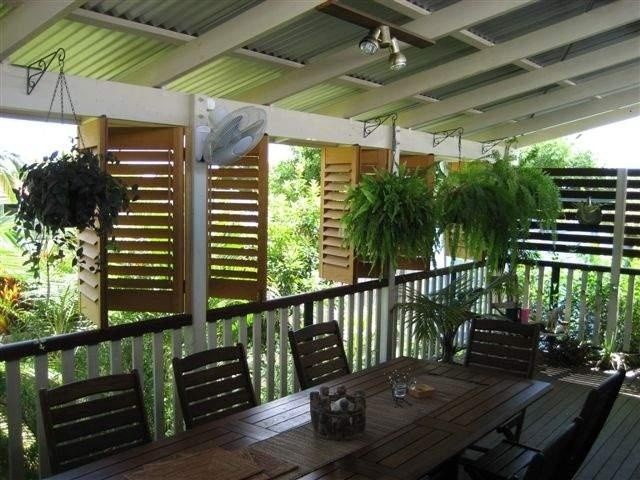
left=199, top=100, right=270, bottom=172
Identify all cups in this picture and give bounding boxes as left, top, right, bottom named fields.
left=391, top=365, right=417, bottom=399
left=521, top=308, right=531, bottom=325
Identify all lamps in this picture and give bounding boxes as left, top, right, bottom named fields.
left=356, top=23, right=410, bottom=74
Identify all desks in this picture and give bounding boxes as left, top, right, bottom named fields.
left=40, top=353, right=555, bottom=480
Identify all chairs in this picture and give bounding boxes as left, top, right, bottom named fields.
left=463, top=364, right=628, bottom=479
left=36, top=365, right=154, bottom=479
left=171, top=339, right=263, bottom=435
left=286, top=317, right=352, bottom=393
left=451, top=313, right=545, bottom=464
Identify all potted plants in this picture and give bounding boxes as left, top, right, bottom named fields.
left=7, top=142, right=144, bottom=287
